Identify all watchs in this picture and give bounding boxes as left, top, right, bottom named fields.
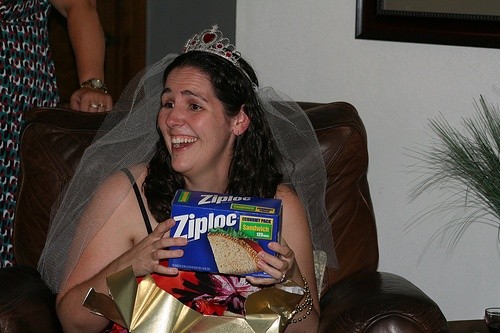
left=81, top=78, right=109, bottom=95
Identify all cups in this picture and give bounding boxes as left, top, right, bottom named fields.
left=483, top=306, right=500, bottom=333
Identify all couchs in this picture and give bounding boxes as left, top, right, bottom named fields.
left=0, top=101, right=454, bottom=333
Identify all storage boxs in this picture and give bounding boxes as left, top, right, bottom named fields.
left=168, top=189, right=283, bottom=278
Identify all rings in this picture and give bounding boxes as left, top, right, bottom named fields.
left=89, top=104, right=99, bottom=109
left=277, top=272, right=286, bottom=283
left=98, top=104, right=107, bottom=108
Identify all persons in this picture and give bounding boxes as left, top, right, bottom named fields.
left=0, top=0, right=112, bottom=333
left=37, top=25, right=321, bottom=333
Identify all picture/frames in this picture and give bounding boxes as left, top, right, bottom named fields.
left=355, top=0, right=500, bottom=49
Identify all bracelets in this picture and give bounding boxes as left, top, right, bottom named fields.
left=288, top=277, right=314, bottom=324
